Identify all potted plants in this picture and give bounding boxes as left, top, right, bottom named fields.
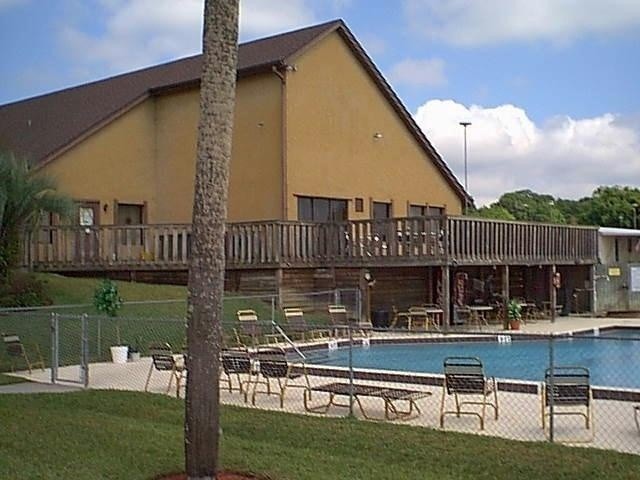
left=92, top=276, right=129, bottom=364
left=116, top=330, right=157, bottom=362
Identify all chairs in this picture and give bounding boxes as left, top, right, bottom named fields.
left=177, top=342, right=232, bottom=400
left=541, top=367, right=595, bottom=443
left=304, top=383, right=432, bottom=422
left=218, top=343, right=270, bottom=403
left=237, top=309, right=288, bottom=345
left=145, top=342, right=184, bottom=395
left=252, top=346, right=312, bottom=408
left=284, top=308, right=333, bottom=342
left=1, top=329, right=46, bottom=375
left=327, top=303, right=374, bottom=339
left=439, top=356, right=498, bottom=430
left=389, top=297, right=563, bottom=333
